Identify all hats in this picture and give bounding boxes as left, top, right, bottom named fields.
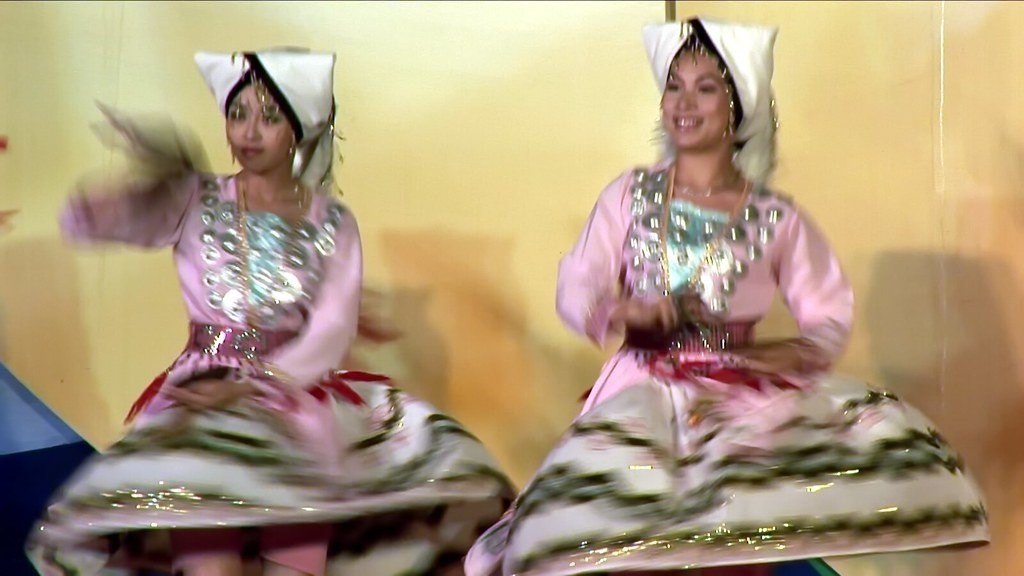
left=643, top=16, right=777, bottom=147
left=193, top=47, right=336, bottom=153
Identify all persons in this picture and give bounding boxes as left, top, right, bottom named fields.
left=22, top=49, right=521, bottom=576
left=463, top=15, right=993, bottom=576
